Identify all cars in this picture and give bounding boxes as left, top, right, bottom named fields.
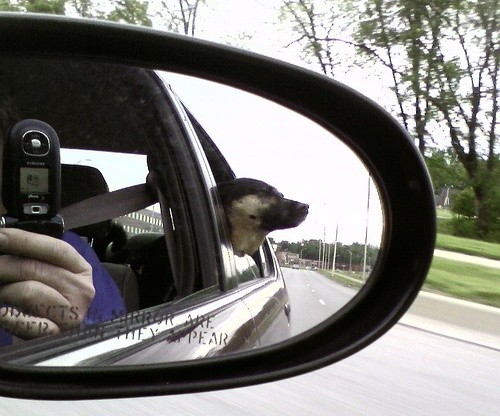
left=0, top=54, right=294, bottom=364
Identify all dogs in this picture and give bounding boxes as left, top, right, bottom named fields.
left=113, top=176, right=312, bottom=308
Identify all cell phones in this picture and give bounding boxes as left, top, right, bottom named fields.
left=6, top=118, right=64, bottom=241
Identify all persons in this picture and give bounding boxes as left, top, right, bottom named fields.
left=0, top=89, right=127, bottom=347
